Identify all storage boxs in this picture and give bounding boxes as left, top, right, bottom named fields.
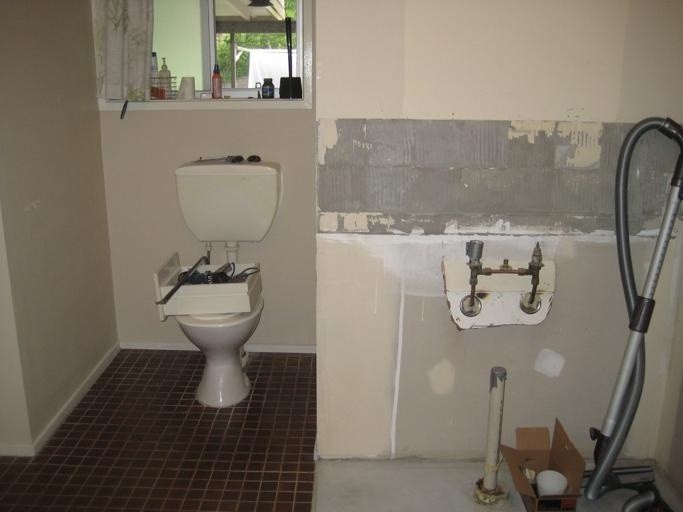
left=499, top=412, right=590, bottom=511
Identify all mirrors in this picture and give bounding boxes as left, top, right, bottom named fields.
left=149, top=0, right=301, bottom=101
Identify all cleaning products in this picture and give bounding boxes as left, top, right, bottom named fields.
left=262, top=77, right=275, bottom=100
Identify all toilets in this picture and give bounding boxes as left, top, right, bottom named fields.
left=169, top=157, right=281, bottom=409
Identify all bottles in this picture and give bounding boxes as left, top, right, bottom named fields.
left=151, top=52, right=158, bottom=88
left=261, top=78, right=274, bottom=99
left=211, top=61, right=222, bottom=99
